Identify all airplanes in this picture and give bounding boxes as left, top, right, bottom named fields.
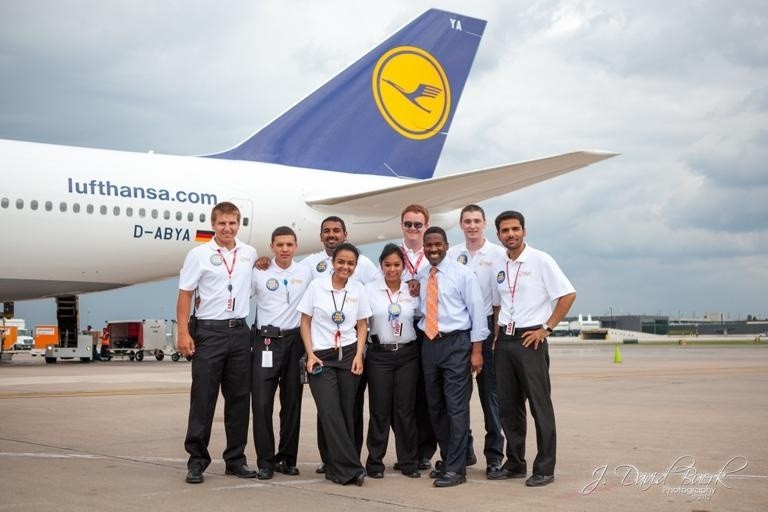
left=0, top=7, right=622, bottom=298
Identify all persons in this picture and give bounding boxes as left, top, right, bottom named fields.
left=88, top=325, right=99, bottom=360
left=99, top=328, right=111, bottom=361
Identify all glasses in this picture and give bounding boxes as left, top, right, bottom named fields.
left=404, top=221, right=422, bottom=228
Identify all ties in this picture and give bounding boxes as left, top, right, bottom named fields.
left=424, top=267, right=438, bottom=340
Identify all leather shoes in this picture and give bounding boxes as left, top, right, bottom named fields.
left=394, top=461, right=431, bottom=477
left=485, top=461, right=526, bottom=479
left=225, top=465, right=256, bottom=478
left=316, top=462, right=327, bottom=473
left=185, top=468, right=203, bottom=483
left=258, top=468, right=272, bottom=479
left=354, top=469, right=383, bottom=485
left=526, top=475, right=554, bottom=486
left=486, top=458, right=502, bottom=472
left=274, top=462, right=298, bottom=475
left=430, top=454, right=477, bottom=486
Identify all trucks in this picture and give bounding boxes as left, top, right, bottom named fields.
left=0, top=317, right=36, bottom=352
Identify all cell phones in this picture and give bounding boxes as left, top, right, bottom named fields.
left=312, top=364, right=322, bottom=374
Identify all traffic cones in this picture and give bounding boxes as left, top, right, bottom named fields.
left=614, top=345, right=623, bottom=364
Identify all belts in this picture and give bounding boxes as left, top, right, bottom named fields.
left=368, top=342, right=414, bottom=351
left=257, top=328, right=300, bottom=339
left=197, top=320, right=245, bottom=328
left=436, top=330, right=468, bottom=338
